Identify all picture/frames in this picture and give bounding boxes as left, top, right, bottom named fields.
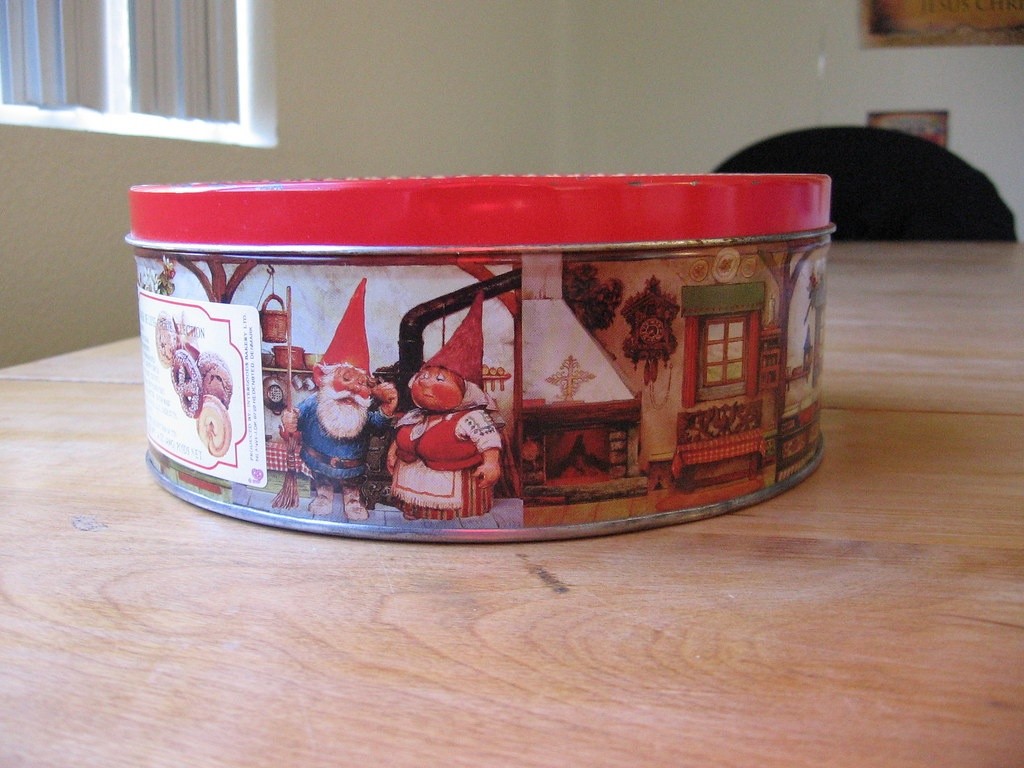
left=868, top=109, right=949, bottom=149
left=857, top=0, right=1024, bottom=47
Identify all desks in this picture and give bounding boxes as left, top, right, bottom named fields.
left=0, top=242, right=1024, bottom=768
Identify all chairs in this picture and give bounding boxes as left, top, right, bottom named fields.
left=712, top=124, right=1017, bottom=245
